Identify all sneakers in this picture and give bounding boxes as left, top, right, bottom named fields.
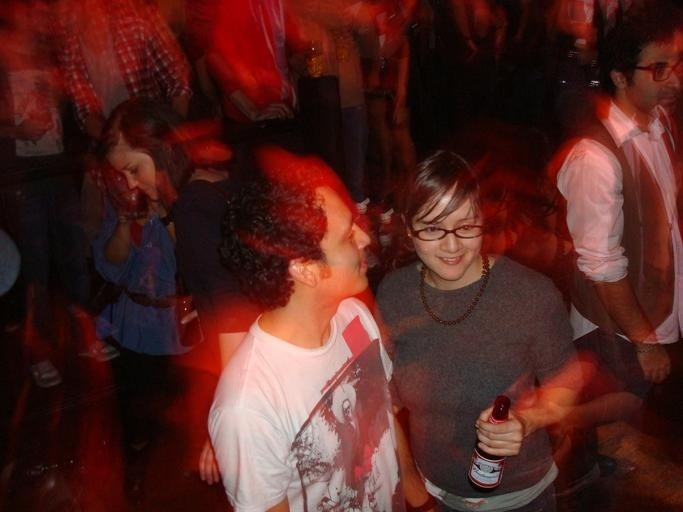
left=124, top=456, right=148, bottom=505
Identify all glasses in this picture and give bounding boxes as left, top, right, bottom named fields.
left=630, top=62, right=683, bottom=82
left=405, top=216, right=488, bottom=242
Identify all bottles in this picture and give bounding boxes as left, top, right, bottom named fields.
left=305, top=38, right=323, bottom=80
left=467, top=395, right=511, bottom=493
